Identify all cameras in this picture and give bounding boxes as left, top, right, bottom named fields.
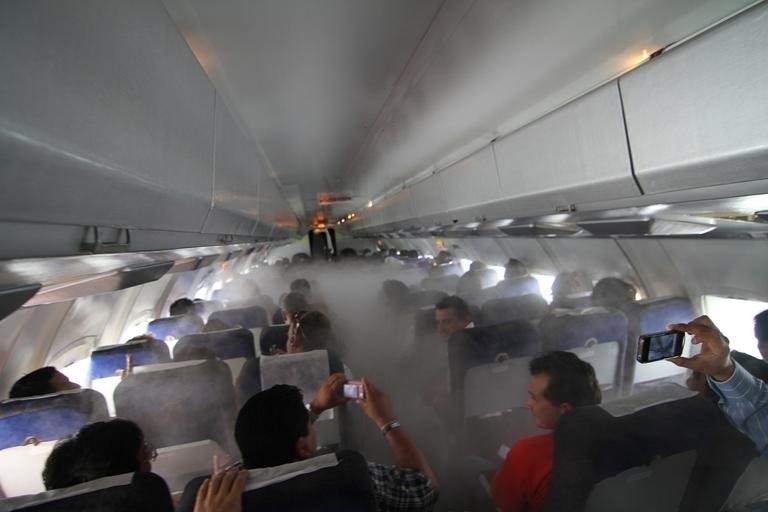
left=342, top=381, right=365, bottom=399
left=637, top=331, right=685, bottom=364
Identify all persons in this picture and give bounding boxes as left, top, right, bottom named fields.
left=179, top=372, right=440, bottom=512
left=493, top=350, right=601, bottom=511
left=377, top=251, right=637, bottom=406
left=667, top=310, right=768, bottom=488
left=42, top=418, right=249, bottom=512
left=127, top=257, right=354, bottom=400
left=8, top=367, right=78, bottom=397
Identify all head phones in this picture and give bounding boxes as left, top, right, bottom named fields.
left=290, top=310, right=315, bottom=346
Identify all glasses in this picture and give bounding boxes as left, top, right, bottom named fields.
left=141, top=443, right=158, bottom=463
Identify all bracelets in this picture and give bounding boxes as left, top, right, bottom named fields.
left=382, top=420, right=401, bottom=435
left=306, top=404, right=321, bottom=419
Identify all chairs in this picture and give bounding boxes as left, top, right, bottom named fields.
left=0, top=248, right=767, bottom=512
